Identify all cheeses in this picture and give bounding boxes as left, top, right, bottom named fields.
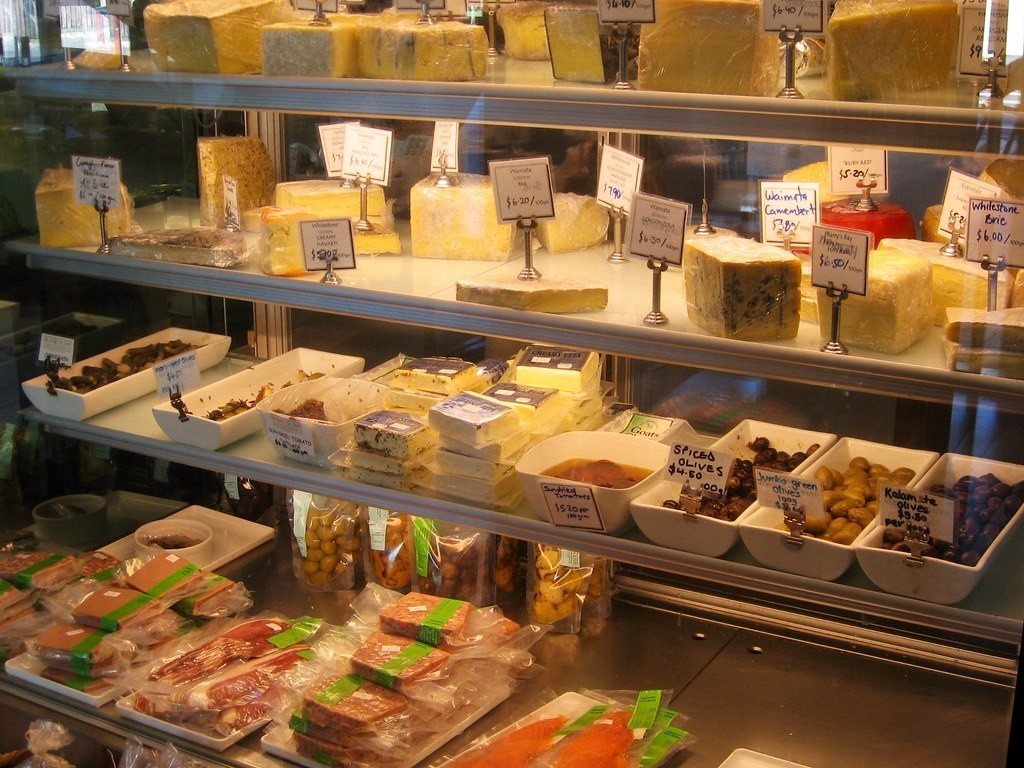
left=33, top=0, right=1024, bottom=483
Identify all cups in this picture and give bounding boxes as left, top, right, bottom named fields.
left=134, top=518, right=211, bottom=568
left=31, top=495, right=108, bottom=546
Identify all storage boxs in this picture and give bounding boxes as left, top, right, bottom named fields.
left=253, top=374, right=387, bottom=466
left=515, top=431, right=671, bottom=531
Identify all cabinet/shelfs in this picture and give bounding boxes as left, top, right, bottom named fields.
left=0, top=71, right=1024, bottom=643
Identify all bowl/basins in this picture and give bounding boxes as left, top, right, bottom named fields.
left=514, top=429, right=670, bottom=535
left=255, top=375, right=389, bottom=470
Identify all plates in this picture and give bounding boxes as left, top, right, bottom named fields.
left=738, top=435, right=941, bottom=581
left=21, top=326, right=232, bottom=421
left=96, top=504, right=274, bottom=571
left=0, top=312, right=128, bottom=380
left=152, top=347, right=365, bottom=450
left=630, top=418, right=835, bottom=557
left=857, top=451, right=1023, bottom=604
left=17, top=490, right=189, bottom=552
